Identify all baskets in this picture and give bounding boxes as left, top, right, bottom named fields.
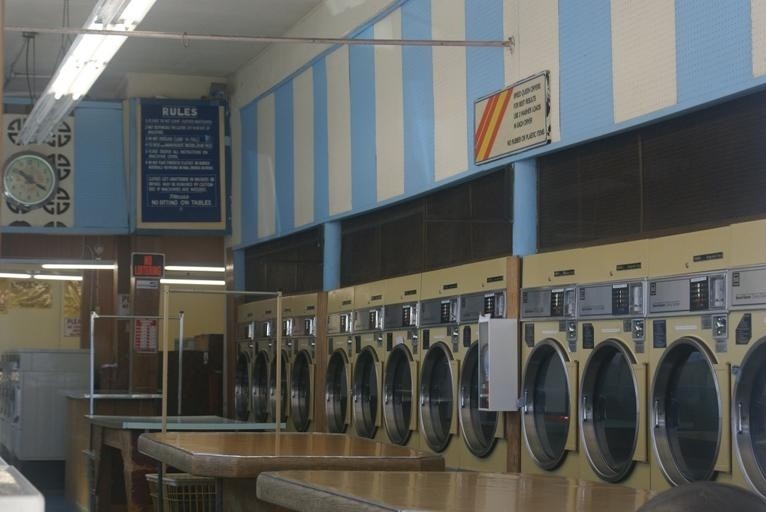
left=145, top=473, right=216, bottom=512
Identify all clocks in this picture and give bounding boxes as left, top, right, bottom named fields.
left=4, top=153, right=56, bottom=206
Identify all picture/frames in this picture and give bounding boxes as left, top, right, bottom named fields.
left=473, top=69, right=551, bottom=166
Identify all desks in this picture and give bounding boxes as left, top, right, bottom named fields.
left=84, top=415, right=288, bottom=512
left=135, top=431, right=447, bottom=512
left=254, top=469, right=667, bottom=511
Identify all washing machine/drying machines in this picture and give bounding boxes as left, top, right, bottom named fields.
left=0, top=348, right=90, bottom=463
left=235, top=218, right=765, bottom=504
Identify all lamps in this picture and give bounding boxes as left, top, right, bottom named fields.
left=17, top=0, right=156, bottom=143
left=0, top=235, right=116, bottom=280
